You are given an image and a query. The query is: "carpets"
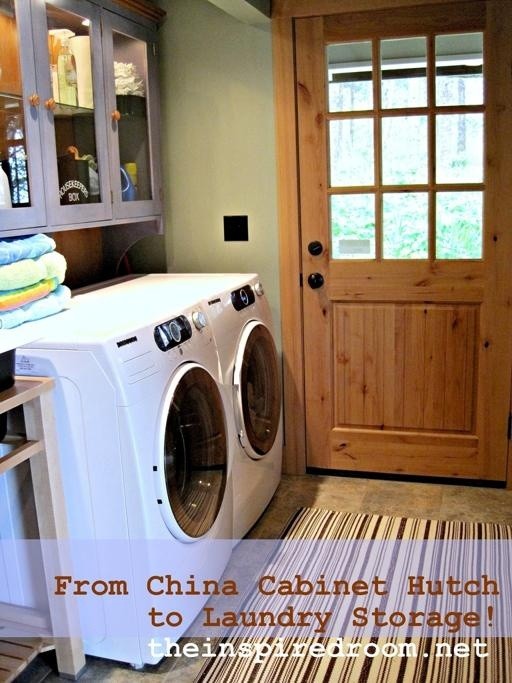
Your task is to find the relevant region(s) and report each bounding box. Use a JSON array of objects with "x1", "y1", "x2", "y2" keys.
[{"x1": 194, "y1": 506, "x2": 512, "y2": 683}]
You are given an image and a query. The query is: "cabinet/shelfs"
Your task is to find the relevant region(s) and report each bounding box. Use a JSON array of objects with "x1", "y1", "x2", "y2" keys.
[{"x1": 0, "y1": 0, "x2": 169, "y2": 238}]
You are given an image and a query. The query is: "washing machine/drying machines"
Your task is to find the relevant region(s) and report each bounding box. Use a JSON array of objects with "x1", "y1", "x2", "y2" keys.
[
  {"x1": 76, "y1": 271, "x2": 285, "y2": 551},
  {"x1": 1, "y1": 298, "x2": 235, "y2": 670}
]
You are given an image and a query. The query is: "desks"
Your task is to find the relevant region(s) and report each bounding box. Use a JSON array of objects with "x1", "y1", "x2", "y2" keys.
[{"x1": 0, "y1": 376, "x2": 88, "y2": 683}]
